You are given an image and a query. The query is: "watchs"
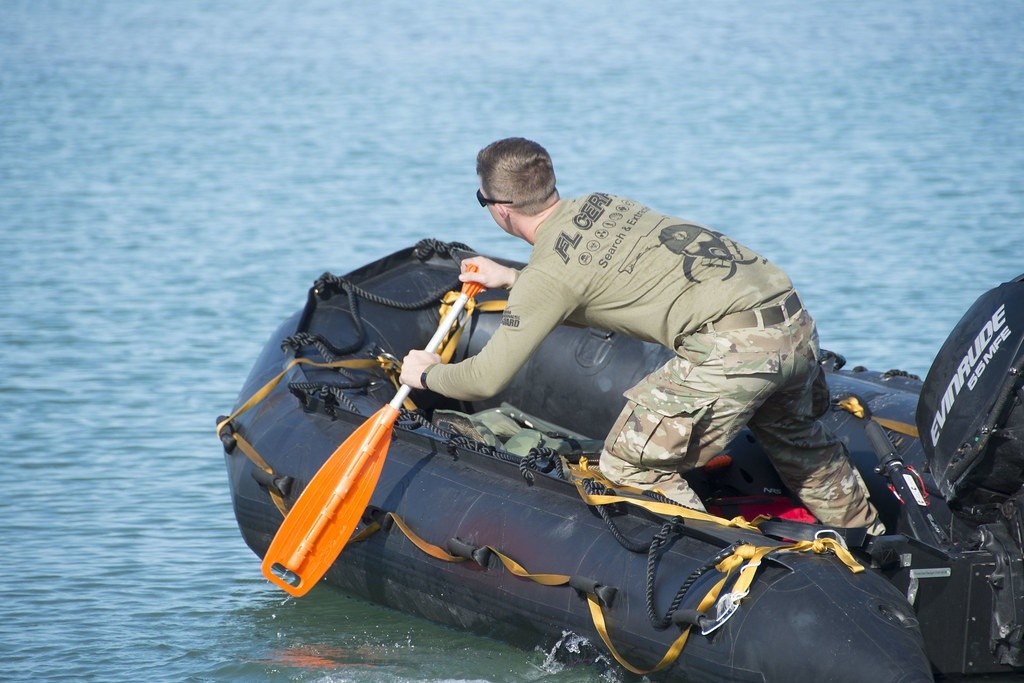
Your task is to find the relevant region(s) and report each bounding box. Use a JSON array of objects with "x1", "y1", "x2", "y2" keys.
[{"x1": 420, "y1": 362, "x2": 443, "y2": 390}]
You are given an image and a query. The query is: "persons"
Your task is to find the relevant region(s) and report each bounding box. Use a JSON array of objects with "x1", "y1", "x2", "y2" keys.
[{"x1": 396, "y1": 135, "x2": 892, "y2": 539}]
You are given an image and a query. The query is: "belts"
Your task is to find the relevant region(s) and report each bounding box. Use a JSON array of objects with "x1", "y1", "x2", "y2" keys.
[{"x1": 697, "y1": 293, "x2": 802, "y2": 334}]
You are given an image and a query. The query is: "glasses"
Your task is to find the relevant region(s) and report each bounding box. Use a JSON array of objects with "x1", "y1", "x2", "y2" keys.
[{"x1": 477, "y1": 189, "x2": 513, "y2": 207}]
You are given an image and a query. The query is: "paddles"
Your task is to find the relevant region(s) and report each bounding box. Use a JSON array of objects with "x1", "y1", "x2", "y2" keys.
[{"x1": 259, "y1": 263, "x2": 485, "y2": 599}]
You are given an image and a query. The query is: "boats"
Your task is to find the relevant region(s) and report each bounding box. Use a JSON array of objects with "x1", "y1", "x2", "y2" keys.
[{"x1": 217, "y1": 238, "x2": 1022, "y2": 683}]
